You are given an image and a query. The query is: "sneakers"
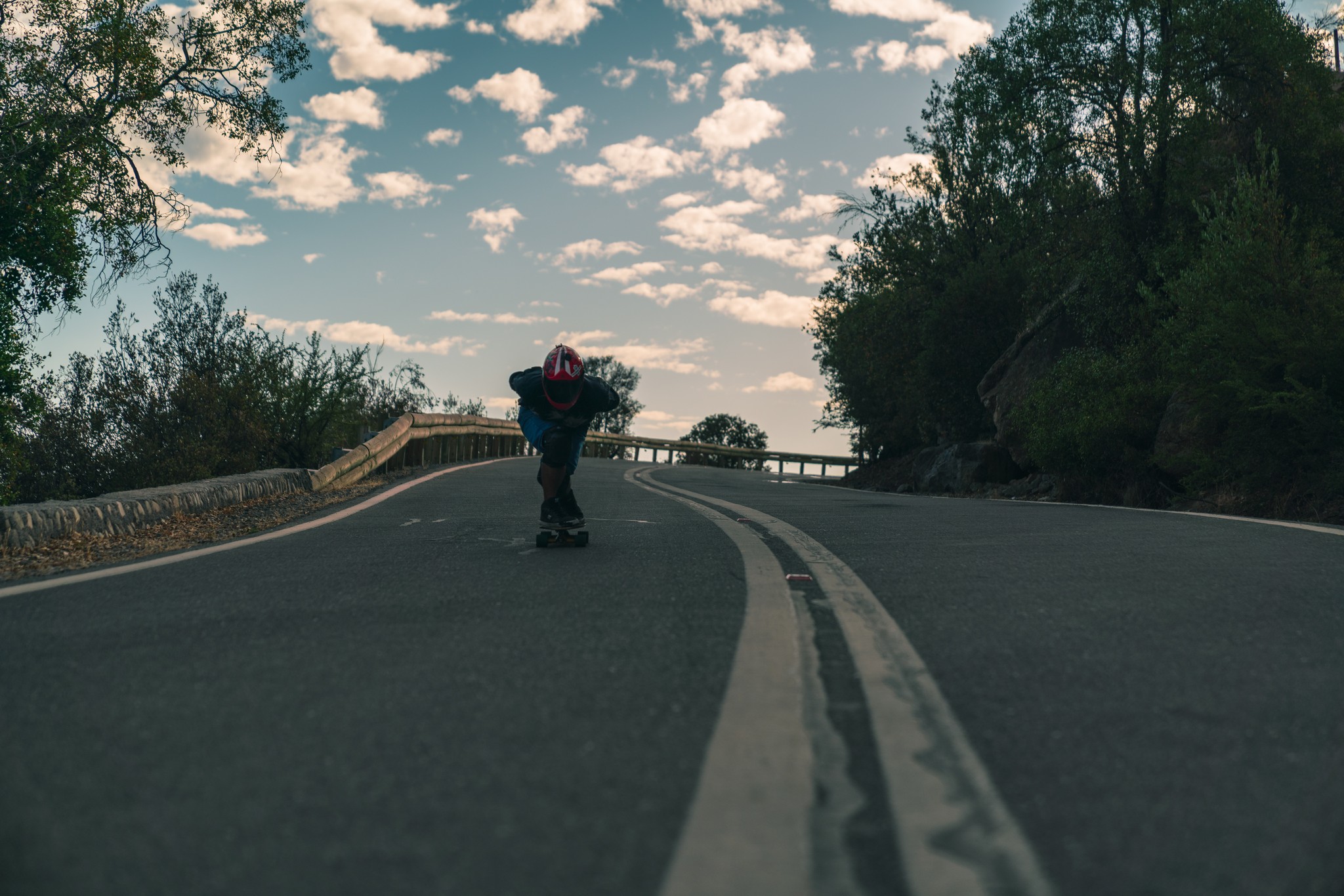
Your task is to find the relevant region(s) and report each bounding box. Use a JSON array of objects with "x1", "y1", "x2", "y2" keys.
[{"x1": 538, "y1": 487, "x2": 587, "y2": 530}]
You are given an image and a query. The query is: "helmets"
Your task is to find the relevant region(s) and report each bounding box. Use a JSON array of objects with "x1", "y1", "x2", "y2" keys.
[{"x1": 543, "y1": 345, "x2": 584, "y2": 379}]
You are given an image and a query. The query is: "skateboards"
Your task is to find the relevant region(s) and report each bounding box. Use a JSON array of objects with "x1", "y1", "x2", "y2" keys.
[{"x1": 536, "y1": 523, "x2": 589, "y2": 547}]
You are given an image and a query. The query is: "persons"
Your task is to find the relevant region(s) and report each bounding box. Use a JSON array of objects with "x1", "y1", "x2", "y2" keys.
[{"x1": 507, "y1": 343, "x2": 620, "y2": 527}]
